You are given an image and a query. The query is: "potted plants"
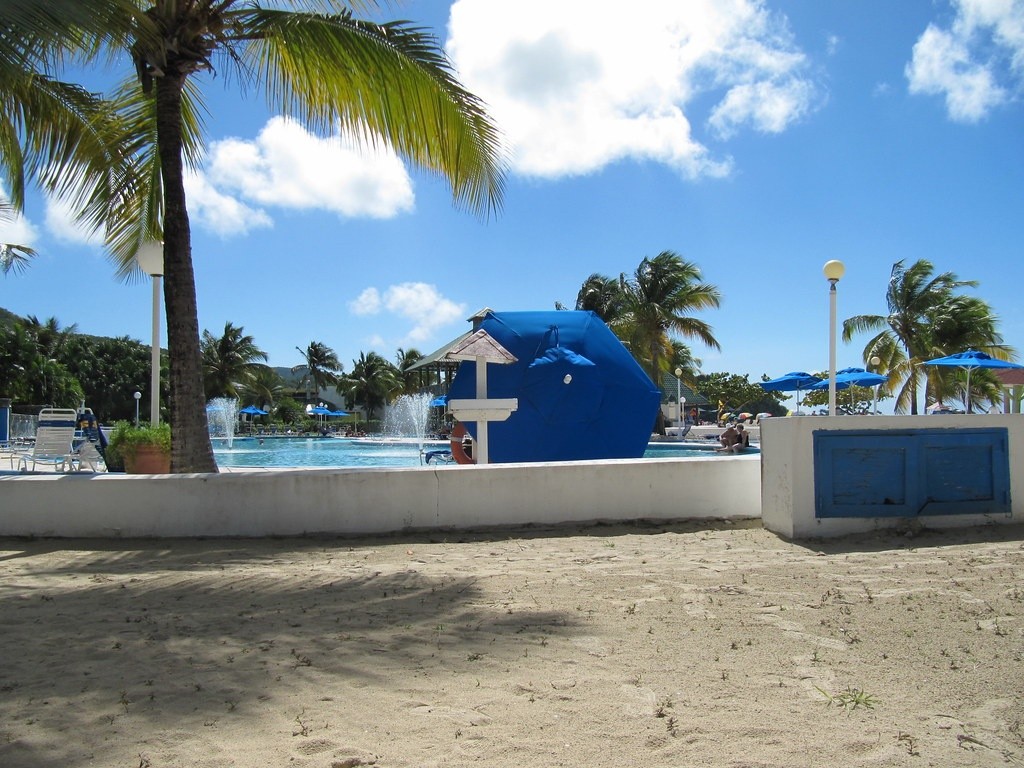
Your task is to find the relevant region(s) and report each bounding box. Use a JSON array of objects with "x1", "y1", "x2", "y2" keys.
[{"x1": 104, "y1": 418, "x2": 171, "y2": 474}]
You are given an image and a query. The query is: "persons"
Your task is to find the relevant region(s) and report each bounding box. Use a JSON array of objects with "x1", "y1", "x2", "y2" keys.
[
  {"x1": 723, "y1": 418, "x2": 753, "y2": 427},
  {"x1": 986, "y1": 402, "x2": 1002, "y2": 414},
  {"x1": 320, "y1": 424, "x2": 364, "y2": 437},
  {"x1": 732, "y1": 424, "x2": 749, "y2": 453},
  {"x1": 439, "y1": 425, "x2": 451, "y2": 434},
  {"x1": 717, "y1": 423, "x2": 738, "y2": 451},
  {"x1": 260, "y1": 438, "x2": 264, "y2": 445},
  {"x1": 700, "y1": 418, "x2": 705, "y2": 425}
]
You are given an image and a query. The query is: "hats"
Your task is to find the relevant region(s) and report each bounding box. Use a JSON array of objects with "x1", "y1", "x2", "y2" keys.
[{"x1": 723, "y1": 423, "x2": 735, "y2": 431}]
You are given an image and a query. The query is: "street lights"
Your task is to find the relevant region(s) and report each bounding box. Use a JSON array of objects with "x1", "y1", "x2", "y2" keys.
[
  {"x1": 680, "y1": 397, "x2": 686, "y2": 426},
  {"x1": 137, "y1": 240, "x2": 164, "y2": 429},
  {"x1": 319, "y1": 402, "x2": 323, "y2": 426},
  {"x1": 871, "y1": 356, "x2": 880, "y2": 415},
  {"x1": 134, "y1": 392, "x2": 141, "y2": 427},
  {"x1": 324, "y1": 404, "x2": 328, "y2": 427},
  {"x1": 823, "y1": 259, "x2": 844, "y2": 416},
  {"x1": 675, "y1": 367, "x2": 682, "y2": 440}
]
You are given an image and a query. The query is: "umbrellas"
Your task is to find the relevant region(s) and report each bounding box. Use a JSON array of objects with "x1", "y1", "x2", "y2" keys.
[
  {"x1": 926, "y1": 401, "x2": 954, "y2": 410},
  {"x1": 720, "y1": 413, "x2": 736, "y2": 421},
  {"x1": 430, "y1": 396, "x2": 447, "y2": 407},
  {"x1": 309, "y1": 406, "x2": 350, "y2": 428},
  {"x1": 758, "y1": 372, "x2": 824, "y2": 415},
  {"x1": 985, "y1": 369, "x2": 1024, "y2": 389},
  {"x1": 813, "y1": 367, "x2": 890, "y2": 415},
  {"x1": 738, "y1": 413, "x2": 753, "y2": 419},
  {"x1": 444, "y1": 311, "x2": 661, "y2": 463},
  {"x1": 914, "y1": 347, "x2": 1024, "y2": 414},
  {"x1": 786, "y1": 410, "x2": 804, "y2": 416},
  {"x1": 756, "y1": 413, "x2": 771, "y2": 420},
  {"x1": 205, "y1": 404, "x2": 269, "y2": 436}
]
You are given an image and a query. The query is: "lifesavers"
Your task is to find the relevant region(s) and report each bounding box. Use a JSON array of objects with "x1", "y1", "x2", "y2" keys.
[{"x1": 451, "y1": 421, "x2": 477, "y2": 464}]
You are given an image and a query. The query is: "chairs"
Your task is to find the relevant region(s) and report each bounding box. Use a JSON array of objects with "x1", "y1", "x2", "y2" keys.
[
  {"x1": 425, "y1": 439, "x2": 472, "y2": 465},
  {"x1": 10, "y1": 408, "x2": 107, "y2": 473},
  {"x1": 668, "y1": 421, "x2": 705, "y2": 441}
]
